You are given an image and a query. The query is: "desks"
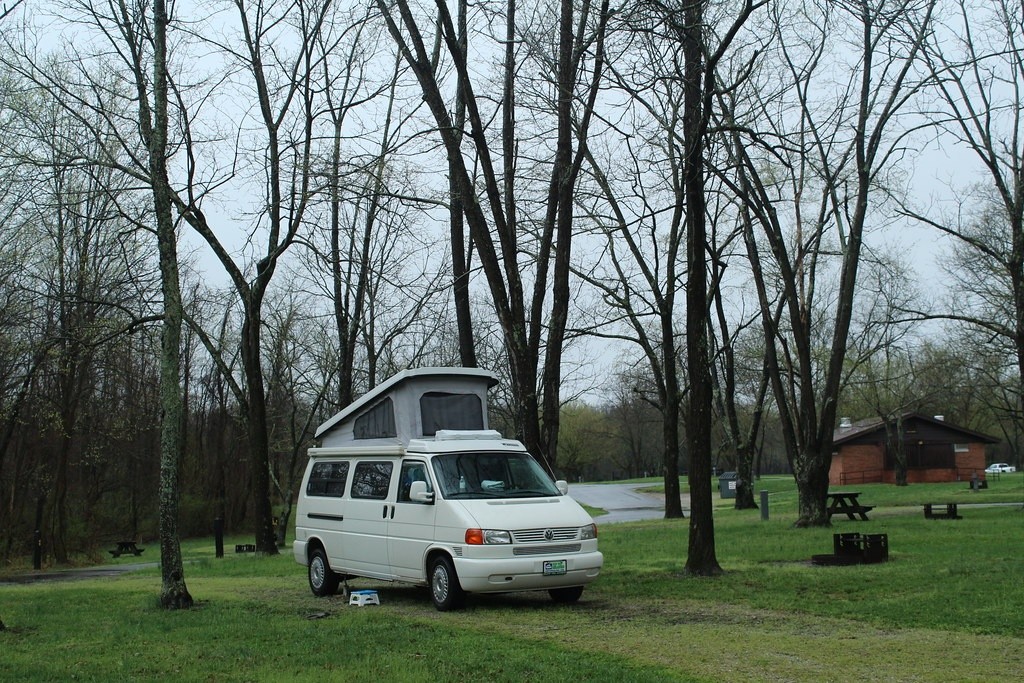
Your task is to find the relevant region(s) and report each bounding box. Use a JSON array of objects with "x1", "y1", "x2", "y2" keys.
[
  {"x1": 827, "y1": 492, "x2": 870, "y2": 521},
  {"x1": 112, "y1": 541, "x2": 142, "y2": 558}
]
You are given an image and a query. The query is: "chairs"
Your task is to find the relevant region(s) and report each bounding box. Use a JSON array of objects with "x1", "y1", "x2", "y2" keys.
[{"x1": 413, "y1": 468, "x2": 426, "y2": 481}]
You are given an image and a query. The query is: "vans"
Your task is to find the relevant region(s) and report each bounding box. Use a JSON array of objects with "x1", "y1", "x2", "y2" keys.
[{"x1": 292, "y1": 366, "x2": 602, "y2": 612}]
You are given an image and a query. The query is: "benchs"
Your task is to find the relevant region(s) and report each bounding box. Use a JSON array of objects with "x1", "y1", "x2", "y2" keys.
[
  {"x1": 137, "y1": 548, "x2": 145, "y2": 553},
  {"x1": 108, "y1": 550, "x2": 116, "y2": 554},
  {"x1": 827, "y1": 505, "x2": 876, "y2": 514}
]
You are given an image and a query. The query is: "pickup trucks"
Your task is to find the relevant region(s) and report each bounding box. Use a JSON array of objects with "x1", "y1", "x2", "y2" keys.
[{"x1": 984, "y1": 463, "x2": 1015, "y2": 473}]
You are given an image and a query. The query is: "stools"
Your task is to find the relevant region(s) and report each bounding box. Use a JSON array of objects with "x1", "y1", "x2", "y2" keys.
[{"x1": 349, "y1": 590, "x2": 381, "y2": 606}]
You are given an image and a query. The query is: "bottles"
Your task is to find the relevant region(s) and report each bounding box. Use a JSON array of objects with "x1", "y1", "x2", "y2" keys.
[{"x1": 459, "y1": 475, "x2": 465, "y2": 493}]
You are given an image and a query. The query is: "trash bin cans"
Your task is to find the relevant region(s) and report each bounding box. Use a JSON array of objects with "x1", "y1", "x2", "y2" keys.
[{"x1": 718, "y1": 471, "x2": 754, "y2": 499}]
